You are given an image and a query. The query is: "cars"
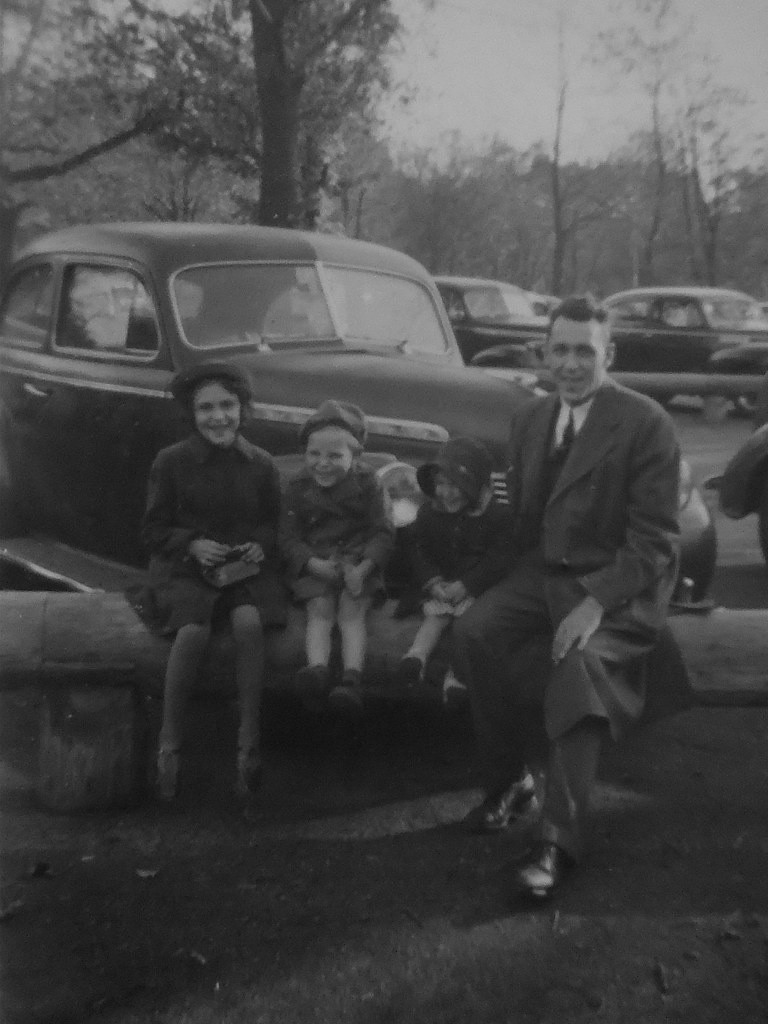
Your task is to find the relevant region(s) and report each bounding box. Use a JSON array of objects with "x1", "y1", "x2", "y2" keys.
[
  {"x1": 429, "y1": 273, "x2": 554, "y2": 368},
  {"x1": 595, "y1": 286, "x2": 768, "y2": 420},
  {"x1": 1, "y1": 221, "x2": 727, "y2": 620}
]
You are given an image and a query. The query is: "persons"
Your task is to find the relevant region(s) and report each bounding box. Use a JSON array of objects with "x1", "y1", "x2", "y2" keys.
[
  {"x1": 399, "y1": 437, "x2": 507, "y2": 712},
  {"x1": 449, "y1": 295, "x2": 682, "y2": 896},
  {"x1": 275, "y1": 400, "x2": 395, "y2": 711},
  {"x1": 124, "y1": 364, "x2": 282, "y2": 797}
]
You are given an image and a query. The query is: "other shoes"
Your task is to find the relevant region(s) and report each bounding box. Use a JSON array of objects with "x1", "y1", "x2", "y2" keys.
[
  {"x1": 232, "y1": 750, "x2": 265, "y2": 790},
  {"x1": 439, "y1": 675, "x2": 468, "y2": 708},
  {"x1": 329, "y1": 677, "x2": 369, "y2": 721},
  {"x1": 149, "y1": 740, "x2": 187, "y2": 797},
  {"x1": 394, "y1": 652, "x2": 427, "y2": 683},
  {"x1": 293, "y1": 666, "x2": 328, "y2": 716}
]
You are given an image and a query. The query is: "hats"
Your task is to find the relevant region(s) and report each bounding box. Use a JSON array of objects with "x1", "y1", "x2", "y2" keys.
[
  {"x1": 301, "y1": 399, "x2": 365, "y2": 445},
  {"x1": 415, "y1": 437, "x2": 493, "y2": 510},
  {"x1": 164, "y1": 358, "x2": 247, "y2": 408}
]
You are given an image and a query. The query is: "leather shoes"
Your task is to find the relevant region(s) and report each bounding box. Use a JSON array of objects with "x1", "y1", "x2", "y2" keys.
[
  {"x1": 510, "y1": 840, "x2": 562, "y2": 891},
  {"x1": 470, "y1": 771, "x2": 537, "y2": 836}
]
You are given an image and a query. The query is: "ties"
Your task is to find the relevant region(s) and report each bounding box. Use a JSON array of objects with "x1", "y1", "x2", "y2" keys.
[{"x1": 538, "y1": 417, "x2": 574, "y2": 527}]
